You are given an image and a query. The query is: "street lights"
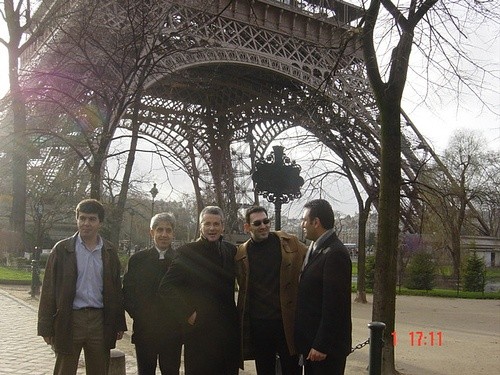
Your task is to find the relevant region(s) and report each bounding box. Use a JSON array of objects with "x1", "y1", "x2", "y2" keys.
[{"x1": 149, "y1": 182, "x2": 159, "y2": 247}]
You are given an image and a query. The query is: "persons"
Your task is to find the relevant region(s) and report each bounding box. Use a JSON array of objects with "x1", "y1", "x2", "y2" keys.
[
  {"x1": 121, "y1": 213, "x2": 182, "y2": 375},
  {"x1": 234, "y1": 207, "x2": 308, "y2": 375},
  {"x1": 158, "y1": 205, "x2": 239, "y2": 374},
  {"x1": 293, "y1": 199, "x2": 353, "y2": 374},
  {"x1": 37, "y1": 200, "x2": 128, "y2": 375}
]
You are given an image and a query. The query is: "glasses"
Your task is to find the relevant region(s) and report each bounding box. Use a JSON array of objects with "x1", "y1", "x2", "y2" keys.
[{"x1": 247, "y1": 219, "x2": 270, "y2": 226}]
